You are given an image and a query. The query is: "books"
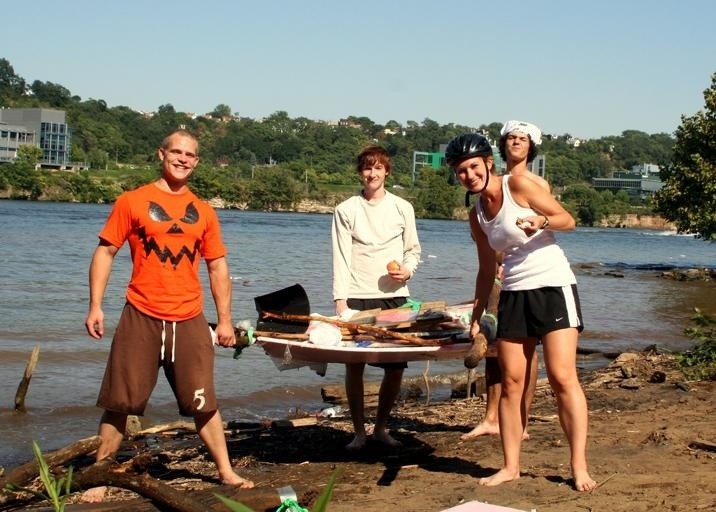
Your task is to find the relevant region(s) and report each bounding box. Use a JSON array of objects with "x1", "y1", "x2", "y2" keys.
[{"x1": 340, "y1": 302, "x2": 475, "y2": 342}]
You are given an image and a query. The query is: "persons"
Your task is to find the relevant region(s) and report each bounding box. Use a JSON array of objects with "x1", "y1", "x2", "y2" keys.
[
  {"x1": 460, "y1": 118, "x2": 553, "y2": 440},
  {"x1": 443, "y1": 132, "x2": 597, "y2": 490},
  {"x1": 81, "y1": 129, "x2": 255, "y2": 502},
  {"x1": 333, "y1": 146, "x2": 421, "y2": 448}
]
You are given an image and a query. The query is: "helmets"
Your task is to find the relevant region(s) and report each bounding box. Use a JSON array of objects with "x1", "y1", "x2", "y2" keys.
[{"x1": 446, "y1": 133, "x2": 492, "y2": 166}]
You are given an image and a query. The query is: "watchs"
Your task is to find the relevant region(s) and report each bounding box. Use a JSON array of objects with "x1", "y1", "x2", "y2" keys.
[{"x1": 539, "y1": 214, "x2": 550, "y2": 230}]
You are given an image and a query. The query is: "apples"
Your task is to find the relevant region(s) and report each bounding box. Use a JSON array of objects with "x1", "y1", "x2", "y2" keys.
[{"x1": 387, "y1": 260, "x2": 399, "y2": 273}]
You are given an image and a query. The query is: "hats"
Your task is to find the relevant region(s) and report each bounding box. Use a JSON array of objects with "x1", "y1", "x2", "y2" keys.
[{"x1": 500, "y1": 121, "x2": 543, "y2": 145}]
